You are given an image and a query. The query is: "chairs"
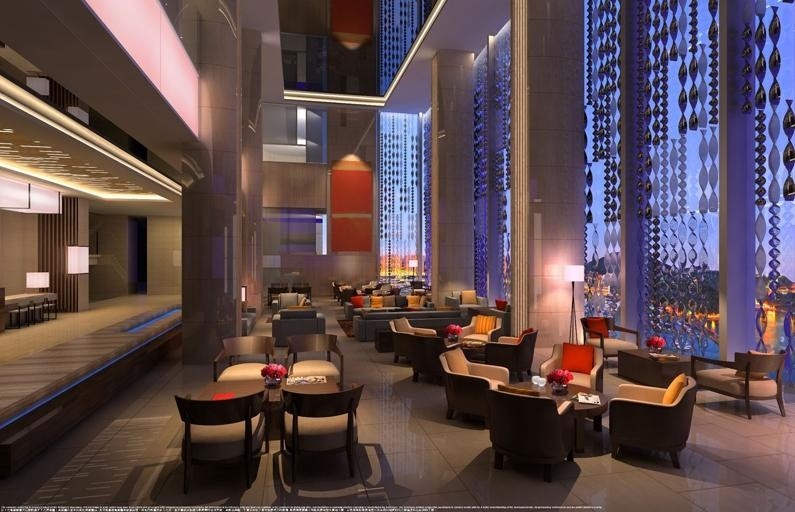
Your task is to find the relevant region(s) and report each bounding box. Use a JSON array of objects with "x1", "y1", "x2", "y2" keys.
[
  {"x1": 30, "y1": 295, "x2": 44, "y2": 324},
  {"x1": 266, "y1": 277, "x2": 537, "y2": 381},
  {"x1": 540, "y1": 343, "x2": 603, "y2": 432},
  {"x1": 44, "y1": 294, "x2": 58, "y2": 320},
  {"x1": 609, "y1": 373, "x2": 697, "y2": 470},
  {"x1": 278, "y1": 385, "x2": 365, "y2": 480},
  {"x1": 692, "y1": 349, "x2": 789, "y2": 419},
  {"x1": 213, "y1": 335, "x2": 273, "y2": 382},
  {"x1": 175, "y1": 392, "x2": 271, "y2": 491},
  {"x1": 284, "y1": 335, "x2": 344, "y2": 386},
  {"x1": 581, "y1": 317, "x2": 640, "y2": 365},
  {"x1": 10, "y1": 297, "x2": 32, "y2": 326},
  {"x1": 440, "y1": 348, "x2": 510, "y2": 427},
  {"x1": 488, "y1": 389, "x2": 574, "y2": 484}
]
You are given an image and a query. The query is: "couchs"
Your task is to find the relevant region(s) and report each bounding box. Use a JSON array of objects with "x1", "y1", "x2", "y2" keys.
[{"x1": 241, "y1": 305, "x2": 257, "y2": 335}]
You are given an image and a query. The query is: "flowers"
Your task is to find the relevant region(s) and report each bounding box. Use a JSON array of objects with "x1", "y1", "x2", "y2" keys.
[
  {"x1": 260, "y1": 363, "x2": 287, "y2": 378},
  {"x1": 547, "y1": 368, "x2": 574, "y2": 384},
  {"x1": 645, "y1": 336, "x2": 666, "y2": 347}
]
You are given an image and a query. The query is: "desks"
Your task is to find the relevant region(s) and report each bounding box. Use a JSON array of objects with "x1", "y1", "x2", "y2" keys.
[
  {"x1": 498, "y1": 383, "x2": 609, "y2": 452},
  {"x1": 0, "y1": 292, "x2": 57, "y2": 330},
  {"x1": 193, "y1": 381, "x2": 355, "y2": 413},
  {"x1": 618, "y1": 348, "x2": 697, "y2": 386}
]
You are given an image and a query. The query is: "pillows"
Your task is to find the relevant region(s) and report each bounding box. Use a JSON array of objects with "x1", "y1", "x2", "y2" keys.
[
  {"x1": 498, "y1": 385, "x2": 540, "y2": 396},
  {"x1": 587, "y1": 318, "x2": 609, "y2": 339},
  {"x1": 562, "y1": 342, "x2": 595, "y2": 374},
  {"x1": 735, "y1": 350, "x2": 776, "y2": 378},
  {"x1": 564, "y1": 265, "x2": 584, "y2": 345},
  {"x1": 663, "y1": 373, "x2": 687, "y2": 405}
]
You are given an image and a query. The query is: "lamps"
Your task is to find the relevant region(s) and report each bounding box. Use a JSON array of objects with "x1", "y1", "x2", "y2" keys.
[
  {"x1": 26, "y1": 272, "x2": 49, "y2": 288},
  {"x1": 240, "y1": 285, "x2": 248, "y2": 303},
  {"x1": 409, "y1": 260, "x2": 419, "y2": 280},
  {"x1": 67, "y1": 245, "x2": 89, "y2": 274},
  {"x1": 0, "y1": 177, "x2": 63, "y2": 215}
]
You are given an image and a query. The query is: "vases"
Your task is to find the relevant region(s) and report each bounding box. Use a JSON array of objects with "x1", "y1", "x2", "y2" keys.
[
  {"x1": 265, "y1": 377, "x2": 283, "y2": 388},
  {"x1": 650, "y1": 346, "x2": 658, "y2": 352},
  {"x1": 551, "y1": 384, "x2": 568, "y2": 395}
]
